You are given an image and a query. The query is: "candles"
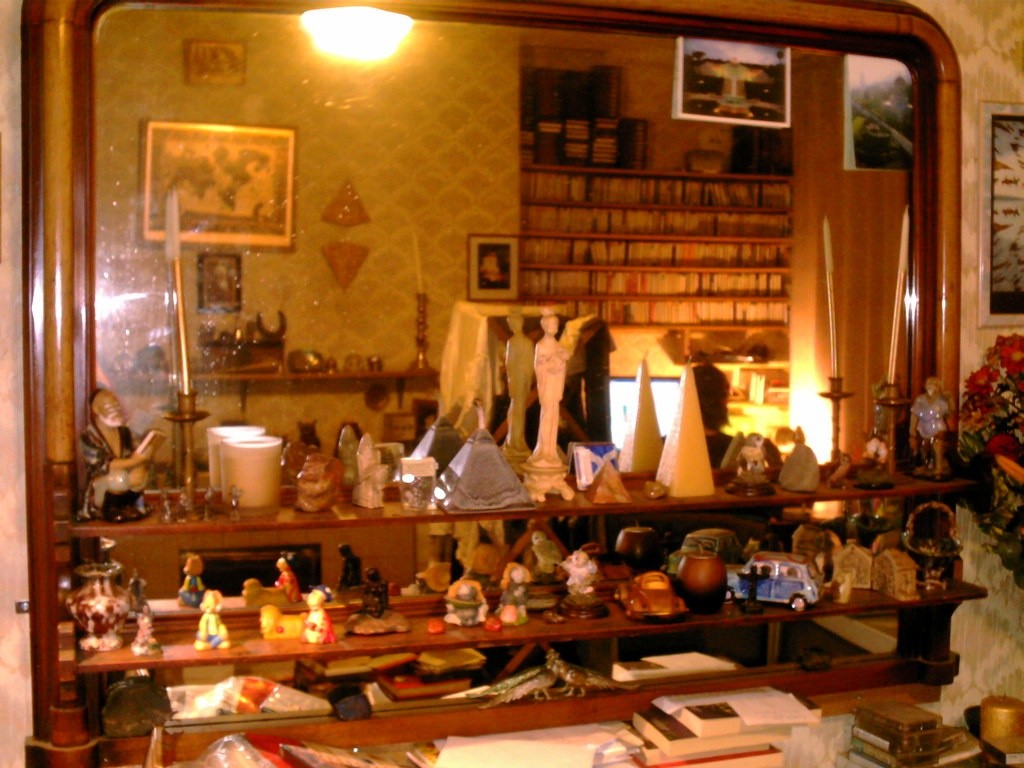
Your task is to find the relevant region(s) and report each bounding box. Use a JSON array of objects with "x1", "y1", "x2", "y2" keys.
[
  {"x1": 886, "y1": 203, "x2": 911, "y2": 386},
  {"x1": 164, "y1": 189, "x2": 180, "y2": 386},
  {"x1": 411, "y1": 225, "x2": 426, "y2": 293},
  {"x1": 172, "y1": 183, "x2": 192, "y2": 394},
  {"x1": 822, "y1": 214, "x2": 843, "y2": 378}
]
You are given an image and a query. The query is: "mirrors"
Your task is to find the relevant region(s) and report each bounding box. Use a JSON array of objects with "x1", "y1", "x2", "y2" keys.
[{"x1": 23, "y1": 0, "x2": 963, "y2": 768}]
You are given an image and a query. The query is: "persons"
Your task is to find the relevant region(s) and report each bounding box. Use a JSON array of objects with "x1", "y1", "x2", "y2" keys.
[
  {"x1": 505, "y1": 314, "x2": 534, "y2": 454},
  {"x1": 337, "y1": 543, "x2": 360, "y2": 591},
  {"x1": 528, "y1": 314, "x2": 568, "y2": 469},
  {"x1": 295, "y1": 454, "x2": 340, "y2": 512},
  {"x1": 355, "y1": 567, "x2": 388, "y2": 617},
  {"x1": 273, "y1": 552, "x2": 303, "y2": 604},
  {"x1": 303, "y1": 584, "x2": 337, "y2": 644},
  {"x1": 662, "y1": 365, "x2": 733, "y2": 469},
  {"x1": 908, "y1": 377, "x2": 956, "y2": 478},
  {"x1": 177, "y1": 554, "x2": 209, "y2": 609},
  {"x1": 193, "y1": 588, "x2": 230, "y2": 650},
  {"x1": 444, "y1": 550, "x2": 598, "y2": 627},
  {"x1": 76, "y1": 387, "x2": 153, "y2": 522}
]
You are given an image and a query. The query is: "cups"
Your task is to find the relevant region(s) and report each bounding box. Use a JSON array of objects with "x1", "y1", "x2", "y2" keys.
[
  {"x1": 220, "y1": 436, "x2": 283, "y2": 516},
  {"x1": 206, "y1": 426, "x2": 266, "y2": 508},
  {"x1": 400, "y1": 457, "x2": 436, "y2": 512}
]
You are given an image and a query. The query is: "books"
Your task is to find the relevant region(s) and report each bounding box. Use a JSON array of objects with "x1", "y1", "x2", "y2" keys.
[
  {"x1": 518, "y1": 67, "x2": 792, "y2": 327},
  {"x1": 618, "y1": 685, "x2": 821, "y2": 767},
  {"x1": 612, "y1": 654, "x2": 740, "y2": 682},
  {"x1": 290, "y1": 647, "x2": 489, "y2": 703}
]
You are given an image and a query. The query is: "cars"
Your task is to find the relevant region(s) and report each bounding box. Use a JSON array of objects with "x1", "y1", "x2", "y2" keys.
[{"x1": 725, "y1": 550, "x2": 824, "y2": 613}]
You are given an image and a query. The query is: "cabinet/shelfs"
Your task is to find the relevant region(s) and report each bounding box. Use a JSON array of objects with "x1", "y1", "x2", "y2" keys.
[
  {"x1": 70, "y1": 477, "x2": 990, "y2": 686},
  {"x1": 511, "y1": 161, "x2": 797, "y2": 331}
]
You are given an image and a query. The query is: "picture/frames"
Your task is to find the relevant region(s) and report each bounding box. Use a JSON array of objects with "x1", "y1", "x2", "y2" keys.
[
  {"x1": 671, "y1": 36, "x2": 794, "y2": 130},
  {"x1": 196, "y1": 252, "x2": 242, "y2": 314},
  {"x1": 466, "y1": 233, "x2": 523, "y2": 302},
  {"x1": 184, "y1": 39, "x2": 248, "y2": 85},
  {"x1": 136, "y1": 117, "x2": 299, "y2": 254},
  {"x1": 978, "y1": 99, "x2": 1024, "y2": 328}
]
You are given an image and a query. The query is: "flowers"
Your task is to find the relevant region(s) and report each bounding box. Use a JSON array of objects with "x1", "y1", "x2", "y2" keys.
[{"x1": 958, "y1": 332, "x2": 1024, "y2": 589}]
